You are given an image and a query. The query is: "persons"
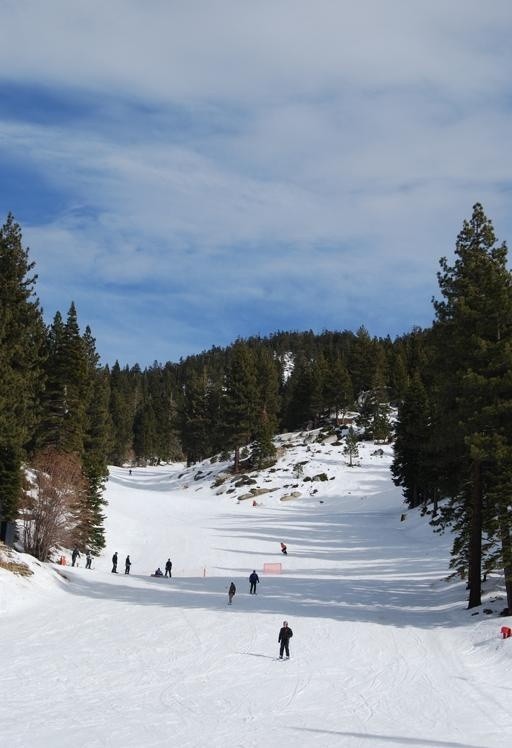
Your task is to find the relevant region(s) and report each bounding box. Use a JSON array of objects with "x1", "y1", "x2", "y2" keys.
[
  {"x1": 250, "y1": 569, "x2": 261, "y2": 595},
  {"x1": 227, "y1": 582, "x2": 238, "y2": 604},
  {"x1": 277, "y1": 620, "x2": 294, "y2": 661},
  {"x1": 281, "y1": 541, "x2": 289, "y2": 556},
  {"x1": 71, "y1": 545, "x2": 174, "y2": 580}
]
